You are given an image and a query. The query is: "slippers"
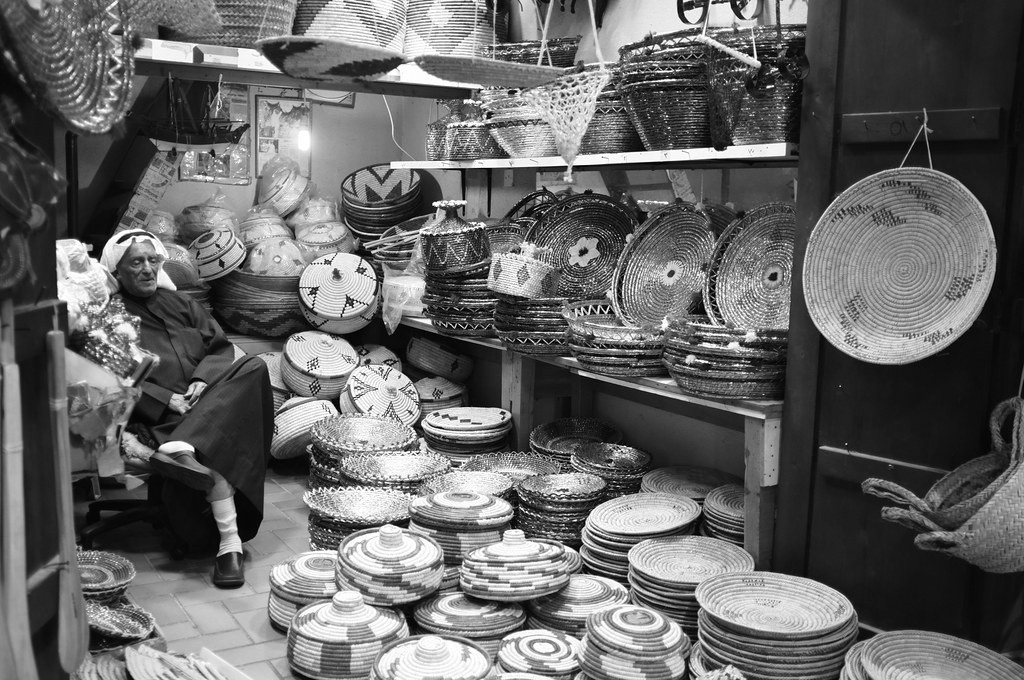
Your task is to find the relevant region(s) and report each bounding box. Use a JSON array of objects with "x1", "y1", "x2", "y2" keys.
[
  {"x1": 213, "y1": 551, "x2": 245, "y2": 586},
  {"x1": 149, "y1": 450, "x2": 215, "y2": 491}
]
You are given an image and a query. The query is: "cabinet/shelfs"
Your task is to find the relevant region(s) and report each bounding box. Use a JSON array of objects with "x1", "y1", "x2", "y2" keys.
[{"x1": 59, "y1": 54, "x2": 799, "y2": 571}]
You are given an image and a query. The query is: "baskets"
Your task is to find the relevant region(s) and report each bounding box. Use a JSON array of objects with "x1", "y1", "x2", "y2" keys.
[{"x1": 1, "y1": 1, "x2": 1024, "y2": 680}]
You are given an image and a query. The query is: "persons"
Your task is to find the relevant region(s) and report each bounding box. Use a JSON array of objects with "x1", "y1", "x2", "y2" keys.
[{"x1": 67, "y1": 228, "x2": 274, "y2": 587}]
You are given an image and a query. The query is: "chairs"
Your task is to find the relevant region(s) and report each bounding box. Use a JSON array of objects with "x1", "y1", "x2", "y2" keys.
[{"x1": 80, "y1": 393, "x2": 218, "y2": 562}]
